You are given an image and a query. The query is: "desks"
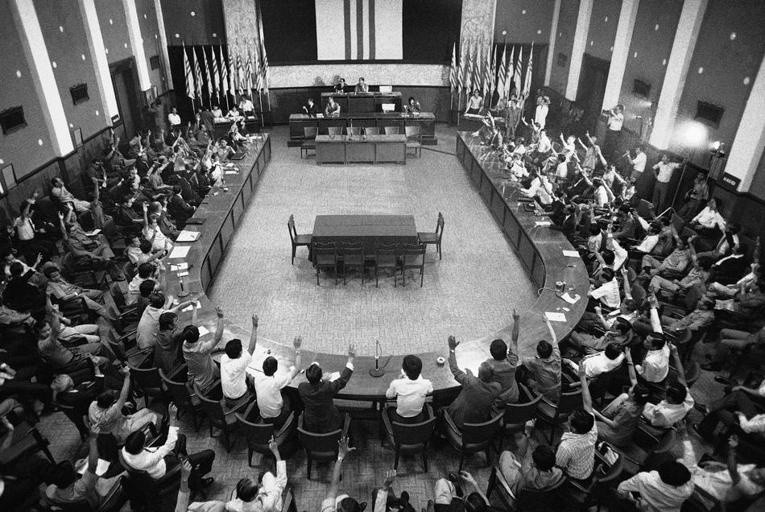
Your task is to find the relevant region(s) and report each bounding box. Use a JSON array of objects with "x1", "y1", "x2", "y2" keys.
[{"x1": 320, "y1": 92, "x2": 402, "y2": 116}]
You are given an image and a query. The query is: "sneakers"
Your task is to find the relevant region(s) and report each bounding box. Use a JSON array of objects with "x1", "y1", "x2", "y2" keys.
[{"x1": 700, "y1": 353, "x2": 721, "y2": 371}]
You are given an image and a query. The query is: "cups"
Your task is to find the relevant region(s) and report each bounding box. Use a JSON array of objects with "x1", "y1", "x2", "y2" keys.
[{"x1": 556, "y1": 282, "x2": 563, "y2": 291}]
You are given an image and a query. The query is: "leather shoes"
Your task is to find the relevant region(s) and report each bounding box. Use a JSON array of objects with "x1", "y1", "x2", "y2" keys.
[
  {"x1": 400, "y1": 491, "x2": 409, "y2": 504},
  {"x1": 694, "y1": 401, "x2": 708, "y2": 417},
  {"x1": 200, "y1": 477, "x2": 214, "y2": 489},
  {"x1": 448, "y1": 473, "x2": 463, "y2": 498},
  {"x1": 358, "y1": 502, "x2": 367, "y2": 512}
]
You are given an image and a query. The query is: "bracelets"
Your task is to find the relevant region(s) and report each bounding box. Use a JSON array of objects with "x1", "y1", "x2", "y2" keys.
[
  {"x1": 296, "y1": 351, "x2": 300, "y2": 354},
  {"x1": 450, "y1": 350, "x2": 454, "y2": 353},
  {"x1": 337, "y1": 457, "x2": 344, "y2": 463}
]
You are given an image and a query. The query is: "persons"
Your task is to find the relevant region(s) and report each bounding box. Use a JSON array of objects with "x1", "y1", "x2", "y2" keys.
[
  {"x1": 225, "y1": 435, "x2": 288, "y2": 512},
  {"x1": 437, "y1": 335, "x2": 502, "y2": 432},
  {"x1": 434, "y1": 469, "x2": 491, "y2": 512},
  {"x1": 372, "y1": 469, "x2": 417, "y2": 512},
  {"x1": 303, "y1": 77, "x2": 369, "y2": 116},
  {"x1": 298, "y1": 343, "x2": 356, "y2": 434},
  {"x1": 386, "y1": 355, "x2": 434, "y2": 424},
  {"x1": 254, "y1": 335, "x2": 303, "y2": 430},
  {"x1": 464, "y1": 89, "x2": 765, "y2": 512},
  {"x1": 0, "y1": 95, "x2": 258, "y2": 512},
  {"x1": 405, "y1": 96, "x2": 421, "y2": 113},
  {"x1": 321, "y1": 435, "x2": 368, "y2": 511}
]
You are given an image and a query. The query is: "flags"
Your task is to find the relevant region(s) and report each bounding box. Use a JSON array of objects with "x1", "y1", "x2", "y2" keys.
[
  {"x1": 183, "y1": 43, "x2": 269, "y2": 98},
  {"x1": 449, "y1": 43, "x2": 533, "y2": 100}
]
